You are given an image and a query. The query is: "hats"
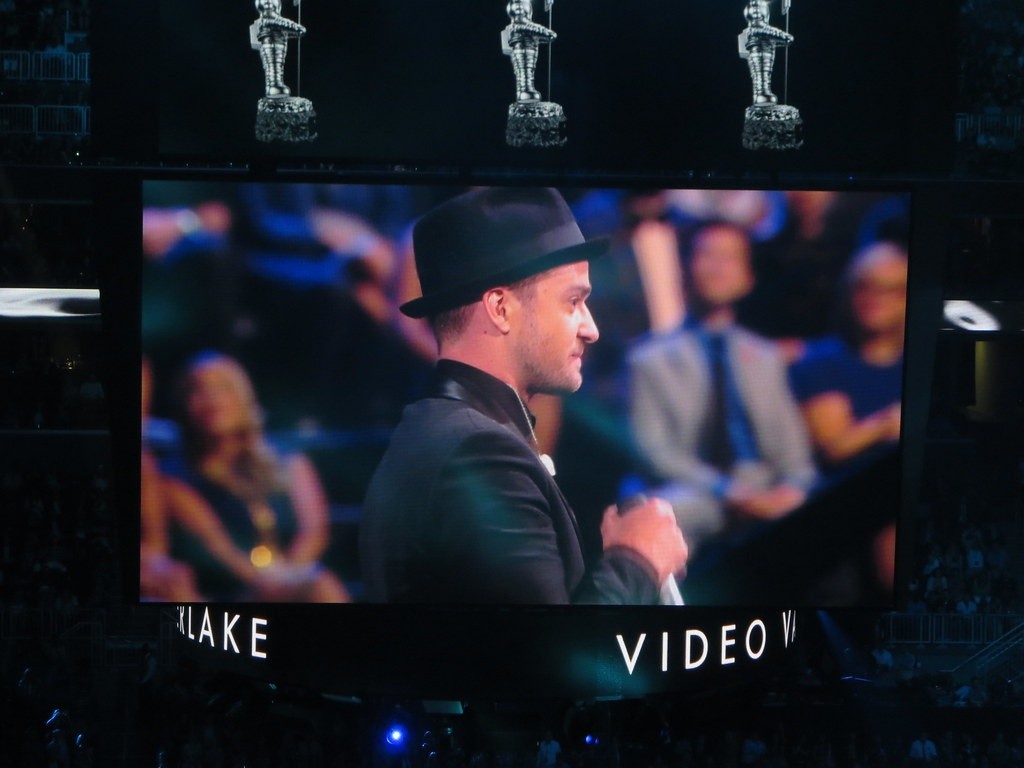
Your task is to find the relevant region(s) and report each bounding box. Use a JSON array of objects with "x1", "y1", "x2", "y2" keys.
[{"x1": 398, "y1": 180, "x2": 613, "y2": 321}]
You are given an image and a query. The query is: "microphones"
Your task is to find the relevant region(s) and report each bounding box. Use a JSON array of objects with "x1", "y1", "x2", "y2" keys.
[{"x1": 621, "y1": 493, "x2": 684, "y2": 607}]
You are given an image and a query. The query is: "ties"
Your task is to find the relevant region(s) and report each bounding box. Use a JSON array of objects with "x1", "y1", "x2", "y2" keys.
[{"x1": 696, "y1": 334, "x2": 739, "y2": 480}]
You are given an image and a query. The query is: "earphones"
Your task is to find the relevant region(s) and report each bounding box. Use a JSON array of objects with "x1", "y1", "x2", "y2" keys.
[{"x1": 540, "y1": 453, "x2": 556, "y2": 477}]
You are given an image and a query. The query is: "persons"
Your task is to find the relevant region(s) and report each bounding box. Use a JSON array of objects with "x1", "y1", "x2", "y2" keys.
[
  {"x1": 942, "y1": 674, "x2": 993, "y2": 703},
  {"x1": 536, "y1": 730, "x2": 562, "y2": 768},
  {"x1": 960, "y1": 128, "x2": 997, "y2": 150},
  {"x1": 914, "y1": 519, "x2": 1016, "y2": 614},
  {"x1": 356, "y1": 186, "x2": 689, "y2": 603},
  {"x1": 142, "y1": 178, "x2": 911, "y2": 604},
  {"x1": 909, "y1": 734, "x2": 937, "y2": 762},
  {"x1": 563, "y1": 698, "x2": 1024, "y2": 768},
  {"x1": 872, "y1": 641, "x2": 915, "y2": 687},
  {"x1": 18, "y1": 641, "x2": 536, "y2": 768},
  {"x1": 741, "y1": 731, "x2": 767, "y2": 764},
  {"x1": 964, "y1": 209, "x2": 991, "y2": 238}
]
